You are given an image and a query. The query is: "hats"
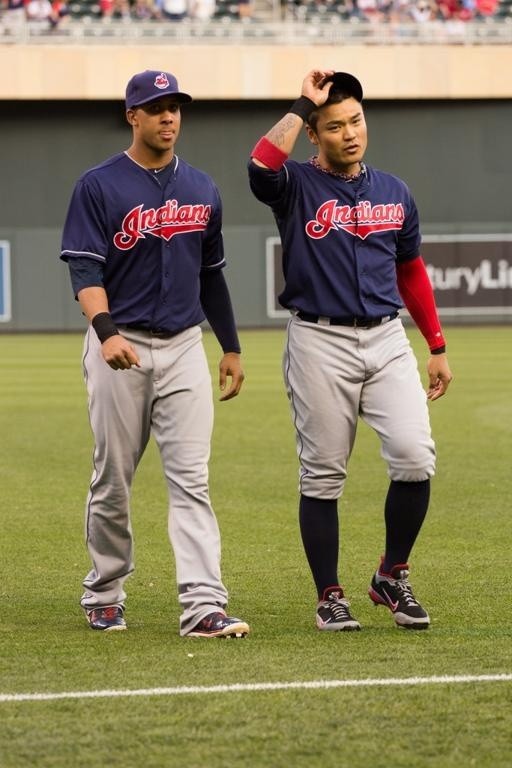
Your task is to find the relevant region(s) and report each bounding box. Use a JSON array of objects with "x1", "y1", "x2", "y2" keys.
[
  {"x1": 125, "y1": 71, "x2": 193, "y2": 111},
  {"x1": 318, "y1": 71, "x2": 363, "y2": 103}
]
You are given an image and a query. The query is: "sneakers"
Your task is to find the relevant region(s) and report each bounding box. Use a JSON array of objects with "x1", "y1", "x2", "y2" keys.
[
  {"x1": 85, "y1": 607, "x2": 127, "y2": 632},
  {"x1": 315, "y1": 585, "x2": 363, "y2": 631},
  {"x1": 185, "y1": 612, "x2": 249, "y2": 639},
  {"x1": 367, "y1": 557, "x2": 431, "y2": 629}
]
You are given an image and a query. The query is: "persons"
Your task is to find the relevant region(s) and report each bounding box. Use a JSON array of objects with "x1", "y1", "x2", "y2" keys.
[
  {"x1": 247, "y1": 68, "x2": 451, "y2": 630},
  {"x1": 60, "y1": 70, "x2": 250, "y2": 639}
]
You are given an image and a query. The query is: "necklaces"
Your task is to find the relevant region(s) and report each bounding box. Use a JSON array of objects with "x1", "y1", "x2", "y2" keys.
[{"x1": 310, "y1": 155, "x2": 367, "y2": 179}]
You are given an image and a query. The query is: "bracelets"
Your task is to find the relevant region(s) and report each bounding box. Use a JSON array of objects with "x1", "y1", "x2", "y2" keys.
[
  {"x1": 288, "y1": 95, "x2": 318, "y2": 123},
  {"x1": 432, "y1": 345, "x2": 446, "y2": 355},
  {"x1": 92, "y1": 313, "x2": 120, "y2": 345}
]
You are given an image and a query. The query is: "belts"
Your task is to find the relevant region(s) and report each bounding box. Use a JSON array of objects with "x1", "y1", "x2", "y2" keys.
[
  {"x1": 297, "y1": 311, "x2": 399, "y2": 326},
  {"x1": 126, "y1": 326, "x2": 168, "y2": 339}
]
